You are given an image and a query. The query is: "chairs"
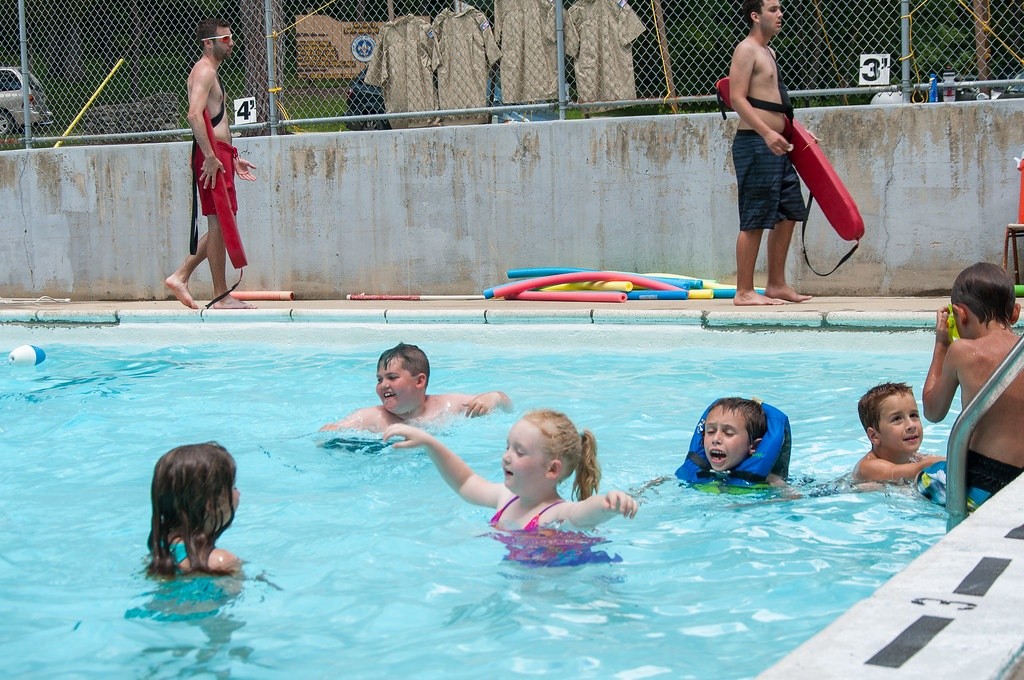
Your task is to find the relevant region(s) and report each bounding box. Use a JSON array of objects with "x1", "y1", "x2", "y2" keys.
[{"x1": 1003, "y1": 156, "x2": 1024, "y2": 282}]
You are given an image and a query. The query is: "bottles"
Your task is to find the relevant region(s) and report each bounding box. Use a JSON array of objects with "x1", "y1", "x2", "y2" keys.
[
  {"x1": 929, "y1": 74, "x2": 938, "y2": 103},
  {"x1": 943, "y1": 65, "x2": 956, "y2": 102}
]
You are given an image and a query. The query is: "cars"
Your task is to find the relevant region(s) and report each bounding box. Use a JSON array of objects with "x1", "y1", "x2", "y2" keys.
[
  {"x1": 344, "y1": 66, "x2": 396, "y2": 130},
  {"x1": 0, "y1": 65, "x2": 53, "y2": 136}
]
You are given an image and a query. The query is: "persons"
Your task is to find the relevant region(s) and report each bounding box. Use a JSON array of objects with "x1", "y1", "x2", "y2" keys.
[
  {"x1": 729, "y1": 0, "x2": 812, "y2": 306},
  {"x1": 146, "y1": 441, "x2": 241, "y2": 575},
  {"x1": 703, "y1": 397, "x2": 784, "y2": 486},
  {"x1": 915, "y1": 261, "x2": 1024, "y2": 512},
  {"x1": 319, "y1": 341, "x2": 515, "y2": 433},
  {"x1": 853, "y1": 381, "x2": 947, "y2": 482},
  {"x1": 383, "y1": 410, "x2": 638, "y2": 533},
  {"x1": 165, "y1": 18, "x2": 258, "y2": 309}
]
